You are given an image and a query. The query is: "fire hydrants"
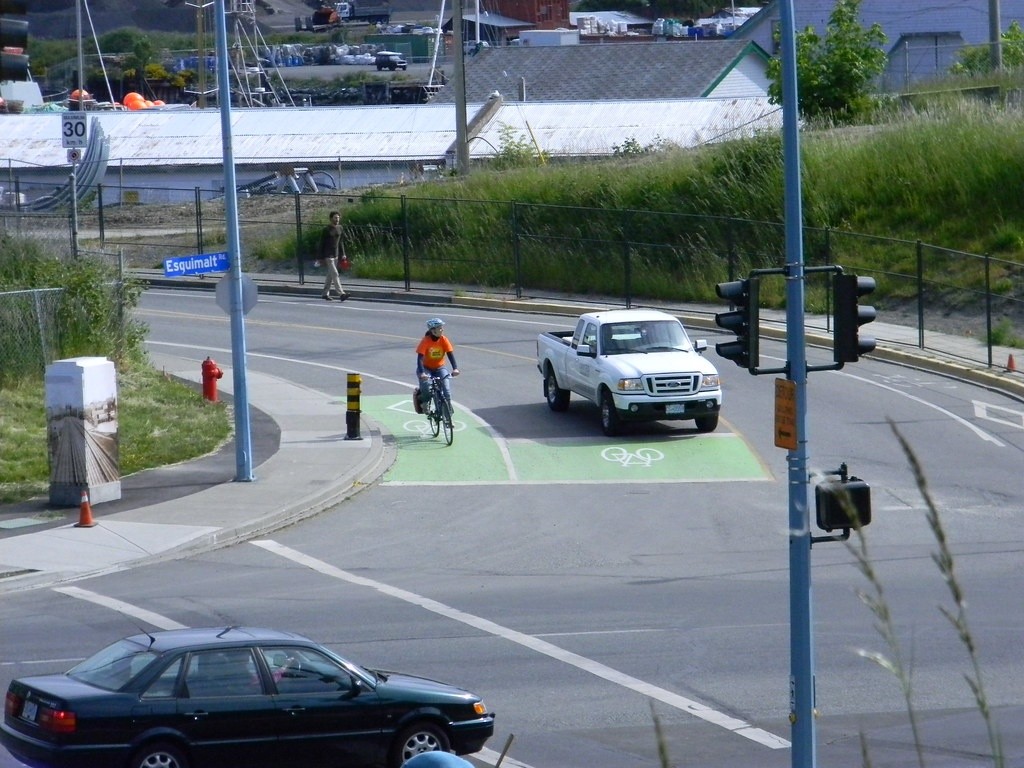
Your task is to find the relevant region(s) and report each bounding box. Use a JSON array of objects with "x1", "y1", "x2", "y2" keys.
[{"x1": 200, "y1": 356, "x2": 224, "y2": 402}]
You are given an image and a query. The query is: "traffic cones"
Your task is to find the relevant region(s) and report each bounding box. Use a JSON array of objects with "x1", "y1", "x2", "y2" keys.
[
  {"x1": 1004, "y1": 353, "x2": 1017, "y2": 373},
  {"x1": 74, "y1": 492, "x2": 99, "y2": 528}
]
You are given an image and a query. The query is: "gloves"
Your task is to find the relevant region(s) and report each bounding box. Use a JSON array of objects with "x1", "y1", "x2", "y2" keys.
[
  {"x1": 420, "y1": 373, "x2": 429, "y2": 382},
  {"x1": 452, "y1": 368, "x2": 459, "y2": 376}
]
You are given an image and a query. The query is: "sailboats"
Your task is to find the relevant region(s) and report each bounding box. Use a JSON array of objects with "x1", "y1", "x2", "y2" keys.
[{"x1": 422, "y1": 0, "x2": 513, "y2": 99}]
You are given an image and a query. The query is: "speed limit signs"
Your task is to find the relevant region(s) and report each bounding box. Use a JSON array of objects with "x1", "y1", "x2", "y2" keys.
[{"x1": 61, "y1": 112, "x2": 89, "y2": 148}]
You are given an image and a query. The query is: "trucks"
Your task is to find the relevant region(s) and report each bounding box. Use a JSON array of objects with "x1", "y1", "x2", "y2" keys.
[{"x1": 334, "y1": 3, "x2": 392, "y2": 24}]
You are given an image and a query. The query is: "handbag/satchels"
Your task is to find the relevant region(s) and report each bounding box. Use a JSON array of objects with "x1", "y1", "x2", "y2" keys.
[{"x1": 414, "y1": 388, "x2": 421, "y2": 413}]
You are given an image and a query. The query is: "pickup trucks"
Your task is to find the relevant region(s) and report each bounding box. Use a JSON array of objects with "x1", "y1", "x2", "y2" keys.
[{"x1": 536, "y1": 308, "x2": 722, "y2": 437}]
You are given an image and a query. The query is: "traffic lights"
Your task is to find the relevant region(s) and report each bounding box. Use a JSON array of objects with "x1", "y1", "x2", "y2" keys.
[
  {"x1": 832, "y1": 273, "x2": 877, "y2": 362},
  {"x1": 714, "y1": 280, "x2": 760, "y2": 367},
  {"x1": 815, "y1": 480, "x2": 872, "y2": 533}
]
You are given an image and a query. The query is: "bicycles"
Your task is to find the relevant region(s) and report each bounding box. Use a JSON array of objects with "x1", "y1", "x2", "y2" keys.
[{"x1": 419, "y1": 373, "x2": 455, "y2": 446}]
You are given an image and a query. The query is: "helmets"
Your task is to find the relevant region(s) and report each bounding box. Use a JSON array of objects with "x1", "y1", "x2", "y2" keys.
[{"x1": 426, "y1": 318, "x2": 445, "y2": 330}]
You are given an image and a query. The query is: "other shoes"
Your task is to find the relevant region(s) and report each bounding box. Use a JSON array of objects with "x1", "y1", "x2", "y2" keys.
[
  {"x1": 420, "y1": 401, "x2": 431, "y2": 415},
  {"x1": 322, "y1": 295, "x2": 333, "y2": 300},
  {"x1": 341, "y1": 294, "x2": 347, "y2": 301}
]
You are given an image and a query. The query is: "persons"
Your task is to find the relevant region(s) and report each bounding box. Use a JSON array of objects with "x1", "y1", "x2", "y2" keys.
[
  {"x1": 415, "y1": 318, "x2": 458, "y2": 428},
  {"x1": 316, "y1": 211, "x2": 350, "y2": 302},
  {"x1": 223, "y1": 652, "x2": 295, "y2": 694}
]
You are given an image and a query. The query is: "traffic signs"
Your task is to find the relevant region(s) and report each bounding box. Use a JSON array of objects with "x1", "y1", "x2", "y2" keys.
[{"x1": 774, "y1": 377, "x2": 798, "y2": 450}]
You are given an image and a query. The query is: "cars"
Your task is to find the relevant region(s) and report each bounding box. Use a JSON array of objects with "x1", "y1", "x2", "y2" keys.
[
  {"x1": 376, "y1": 52, "x2": 408, "y2": 71},
  {"x1": 0, "y1": 625, "x2": 496, "y2": 768},
  {"x1": 464, "y1": 41, "x2": 488, "y2": 54}
]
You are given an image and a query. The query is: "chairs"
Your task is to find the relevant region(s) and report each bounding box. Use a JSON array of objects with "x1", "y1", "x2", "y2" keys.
[
  {"x1": 595, "y1": 325, "x2": 619, "y2": 352},
  {"x1": 198, "y1": 653, "x2": 249, "y2": 692},
  {"x1": 112, "y1": 656, "x2": 131, "y2": 680}
]
